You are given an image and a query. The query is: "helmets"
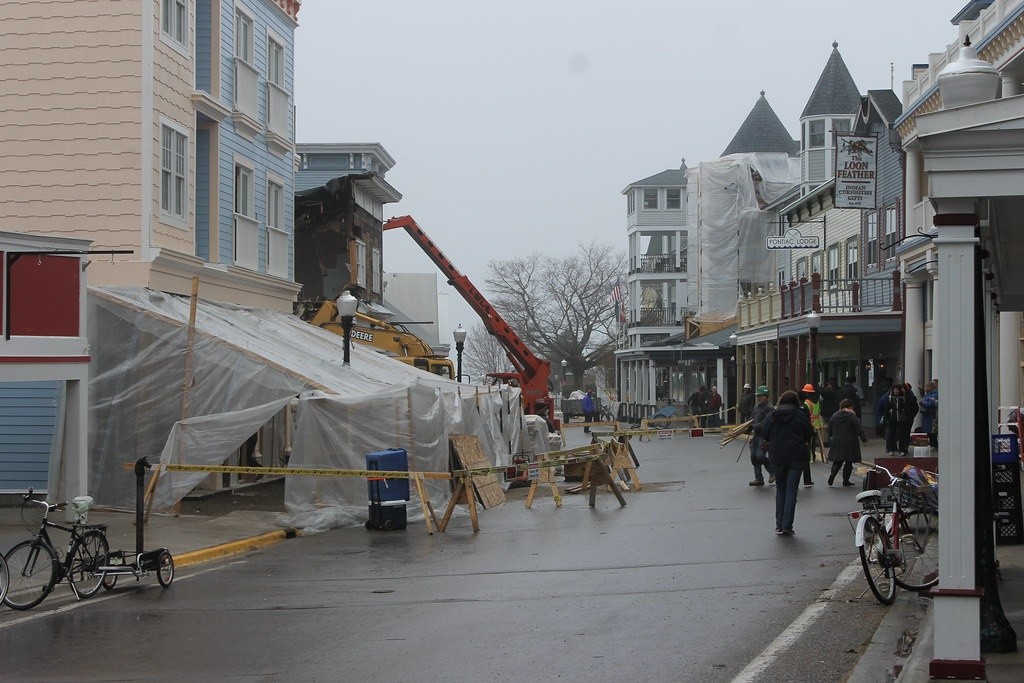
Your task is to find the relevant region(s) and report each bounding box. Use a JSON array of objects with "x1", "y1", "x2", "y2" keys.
[
  {"x1": 802, "y1": 383, "x2": 815, "y2": 393},
  {"x1": 743, "y1": 384, "x2": 751, "y2": 389},
  {"x1": 754, "y1": 385, "x2": 769, "y2": 395}
]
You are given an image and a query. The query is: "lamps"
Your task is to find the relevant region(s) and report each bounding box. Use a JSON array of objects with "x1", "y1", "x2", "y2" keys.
[{"x1": 936, "y1": 33, "x2": 999, "y2": 109}]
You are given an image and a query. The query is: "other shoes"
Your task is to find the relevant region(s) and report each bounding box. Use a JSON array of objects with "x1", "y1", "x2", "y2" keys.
[
  {"x1": 769, "y1": 472, "x2": 776, "y2": 482},
  {"x1": 843, "y1": 480, "x2": 855, "y2": 486},
  {"x1": 890, "y1": 451, "x2": 894, "y2": 456},
  {"x1": 828, "y1": 473, "x2": 834, "y2": 486},
  {"x1": 803, "y1": 481, "x2": 814, "y2": 485},
  {"x1": 899, "y1": 452, "x2": 906, "y2": 456},
  {"x1": 775, "y1": 528, "x2": 795, "y2": 535},
  {"x1": 749, "y1": 478, "x2": 764, "y2": 486}
]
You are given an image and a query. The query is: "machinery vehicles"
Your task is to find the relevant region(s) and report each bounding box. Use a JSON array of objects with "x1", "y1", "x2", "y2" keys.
[
  {"x1": 384, "y1": 215, "x2": 561, "y2": 433},
  {"x1": 561, "y1": 384, "x2": 601, "y2": 423},
  {"x1": 293, "y1": 296, "x2": 455, "y2": 380}
]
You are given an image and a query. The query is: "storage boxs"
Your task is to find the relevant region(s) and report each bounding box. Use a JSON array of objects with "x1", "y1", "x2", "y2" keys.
[
  {"x1": 991, "y1": 434, "x2": 1024, "y2": 545},
  {"x1": 366, "y1": 448, "x2": 409, "y2": 502},
  {"x1": 366, "y1": 501, "x2": 406, "y2": 529}
]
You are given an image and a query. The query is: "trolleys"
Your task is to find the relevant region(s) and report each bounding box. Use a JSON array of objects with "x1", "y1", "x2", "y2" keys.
[
  {"x1": 648, "y1": 401, "x2": 687, "y2": 430},
  {"x1": 365, "y1": 460, "x2": 407, "y2": 531}
]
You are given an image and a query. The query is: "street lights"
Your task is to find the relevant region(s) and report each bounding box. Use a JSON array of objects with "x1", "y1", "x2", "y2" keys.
[
  {"x1": 554, "y1": 374, "x2": 559, "y2": 406},
  {"x1": 806, "y1": 310, "x2": 820, "y2": 388},
  {"x1": 728, "y1": 331, "x2": 737, "y2": 423},
  {"x1": 336, "y1": 290, "x2": 358, "y2": 362},
  {"x1": 453, "y1": 323, "x2": 467, "y2": 382},
  {"x1": 510, "y1": 363, "x2": 515, "y2": 373},
  {"x1": 561, "y1": 358, "x2": 567, "y2": 381}
]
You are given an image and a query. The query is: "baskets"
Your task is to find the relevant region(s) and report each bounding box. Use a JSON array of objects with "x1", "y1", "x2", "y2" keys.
[{"x1": 892, "y1": 471, "x2": 939, "y2": 512}]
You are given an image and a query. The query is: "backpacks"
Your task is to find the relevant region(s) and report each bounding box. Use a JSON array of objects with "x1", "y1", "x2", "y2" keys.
[{"x1": 901, "y1": 464, "x2": 939, "y2": 509}]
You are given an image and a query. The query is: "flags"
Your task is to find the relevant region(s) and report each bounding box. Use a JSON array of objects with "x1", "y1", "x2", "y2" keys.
[{"x1": 608, "y1": 282, "x2": 627, "y2": 323}]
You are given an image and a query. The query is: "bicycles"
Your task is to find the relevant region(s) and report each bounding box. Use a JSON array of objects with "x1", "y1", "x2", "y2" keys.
[
  {"x1": 0, "y1": 487, "x2": 174, "y2": 610},
  {"x1": 0, "y1": 552, "x2": 10, "y2": 605},
  {"x1": 847, "y1": 461, "x2": 939, "y2": 605},
  {"x1": 599, "y1": 404, "x2": 615, "y2": 421}
]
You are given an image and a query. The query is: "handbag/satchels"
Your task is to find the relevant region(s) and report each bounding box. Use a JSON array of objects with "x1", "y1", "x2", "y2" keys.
[
  {"x1": 897, "y1": 409, "x2": 908, "y2": 423},
  {"x1": 919, "y1": 408, "x2": 929, "y2": 415},
  {"x1": 932, "y1": 401, "x2": 939, "y2": 434},
  {"x1": 879, "y1": 416, "x2": 886, "y2": 425}
]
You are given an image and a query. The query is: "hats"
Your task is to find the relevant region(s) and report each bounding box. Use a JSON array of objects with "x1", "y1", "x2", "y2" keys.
[
  {"x1": 903, "y1": 382, "x2": 911, "y2": 393},
  {"x1": 826, "y1": 380, "x2": 835, "y2": 386}
]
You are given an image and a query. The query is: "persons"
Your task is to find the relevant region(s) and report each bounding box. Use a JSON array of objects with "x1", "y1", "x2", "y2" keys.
[
  {"x1": 743, "y1": 386, "x2": 776, "y2": 486},
  {"x1": 737, "y1": 383, "x2": 755, "y2": 423},
  {"x1": 582, "y1": 390, "x2": 595, "y2": 432},
  {"x1": 797, "y1": 375, "x2": 938, "y2": 487},
  {"x1": 762, "y1": 391, "x2": 814, "y2": 535},
  {"x1": 687, "y1": 385, "x2": 723, "y2": 427}
]
